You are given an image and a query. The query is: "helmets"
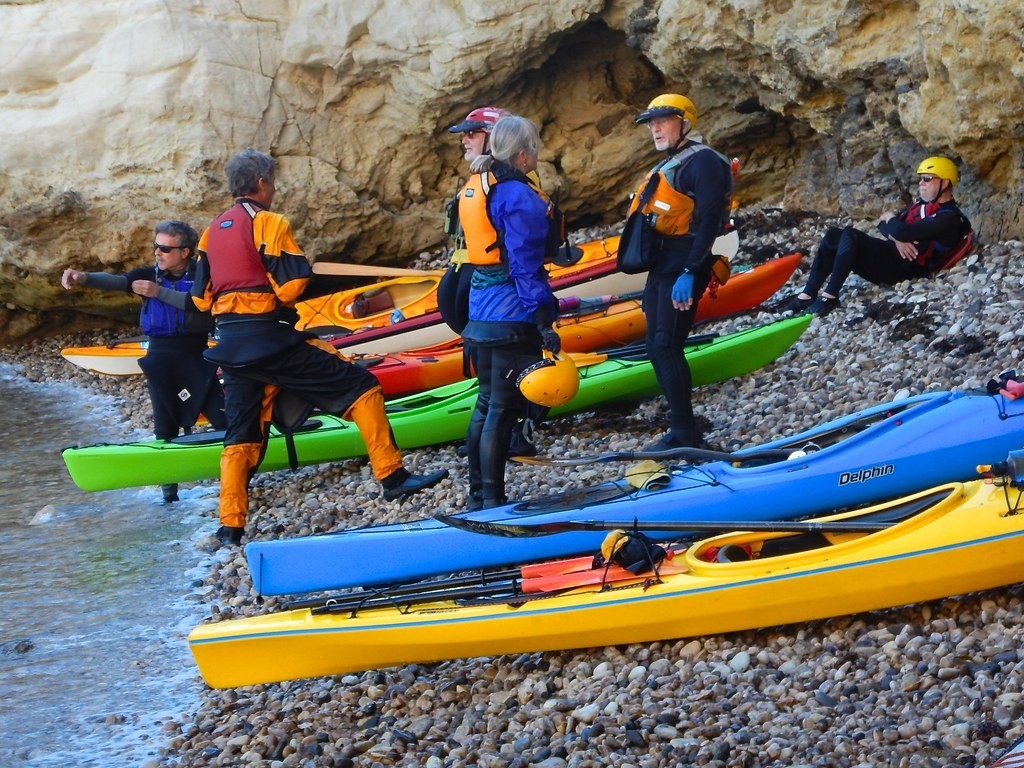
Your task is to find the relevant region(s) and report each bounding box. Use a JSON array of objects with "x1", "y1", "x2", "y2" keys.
[
  {"x1": 447, "y1": 107, "x2": 510, "y2": 133},
  {"x1": 634, "y1": 93, "x2": 698, "y2": 130},
  {"x1": 917, "y1": 156, "x2": 958, "y2": 187},
  {"x1": 516, "y1": 348, "x2": 580, "y2": 407}
]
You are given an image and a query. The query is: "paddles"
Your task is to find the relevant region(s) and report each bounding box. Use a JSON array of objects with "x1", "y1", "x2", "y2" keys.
[
  {"x1": 313, "y1": 261, "x2": 446, "y2": 277},
  {"x1": 433, "y1": 511, "x2": 896, "y2": 536},
  {"x1": 565, "y1": 335, "x2": 720, "y2": 366},
  {"x1": 509, "y1": 446, "x2": 803, "y2": 467},
  {"x1": 285, "y1": 553, "x2": 689, "y2": 613}
]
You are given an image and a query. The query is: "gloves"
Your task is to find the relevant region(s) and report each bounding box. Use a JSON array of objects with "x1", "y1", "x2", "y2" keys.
[{"x1": 540, "y1": 325, "x2": 561, "y2": 354}]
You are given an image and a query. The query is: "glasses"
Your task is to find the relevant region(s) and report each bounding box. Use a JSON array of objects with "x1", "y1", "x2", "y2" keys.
[
  {"x1": 648, "y1": 115, "x2": 680, "y2": 129},
  {"x1": 918, "y1": 174, "x2": 942, "y2": 182},
  {"x1": 463, "y1": 129, "x2": 486, "y2": 139},
  {"x1": 153, "y1": 242, "x2": 187, "y2": 253}
]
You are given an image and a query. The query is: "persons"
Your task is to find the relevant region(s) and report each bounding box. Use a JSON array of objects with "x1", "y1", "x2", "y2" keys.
[
  {"x1": 627, "y1": 93, "x2": 735, "y2": 452},
  {"x1": 62, "y1": 219, "x2": 225, "y2": 442},
  {"x1": 190, "y1": 149, "x2": 450, "y2": 548},
  {"x1": 776, "y1": 156, "x2": 974, "y2": 319},
  {"x1": 458, "y1": 115, "x2": 562, "y2": 512},
  {"x1": 449, "y1": 107, "x2": 541, "y2": 460}
]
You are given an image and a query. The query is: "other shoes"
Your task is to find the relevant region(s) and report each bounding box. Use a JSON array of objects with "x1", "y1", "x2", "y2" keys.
[
  {"x1": 164, "y1": 494, "x2": 179, "y2": 503},
  {"x1": 382, "y1": 466, "x2": 450, "y2": 502},
  {"x1": 771, "y1": 295, "x2": 815, "y2": 313},
  {"x1": 798, "y1": 296, "x2": 841, "y2": 316},
  {"x1": 467, "y1": 495, "x2": 515, "y2": 511},
  {"x1": 458, "y1": 425, "x2": 536, "y2": 462},
  {"x1": 642, "y1": 432, "x2": 709, "y2": 460},
  {"x1": 216, "y1": 526, "x2": 245, "y2": 548}
]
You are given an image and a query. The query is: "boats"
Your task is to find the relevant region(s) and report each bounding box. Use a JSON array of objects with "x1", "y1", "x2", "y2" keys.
[
  {"x1": 356, "y1": 247, "x2": 810, "y2": 398},
  {"x1": 55, "y1": 308, "x2": 819, "y2": 495},
  {"x1": 237, "y1": 363, "x2": 1024, "y2": 605},
  {"x1": 209, "y1": 210, "x2": 747, "y2": 376},
  {"x1": 60, "y1": 156, "x2": 743, "y2": 375},
  {"x1": 187, "y1": 443, "x2": 1024, "y2": 693}
]
